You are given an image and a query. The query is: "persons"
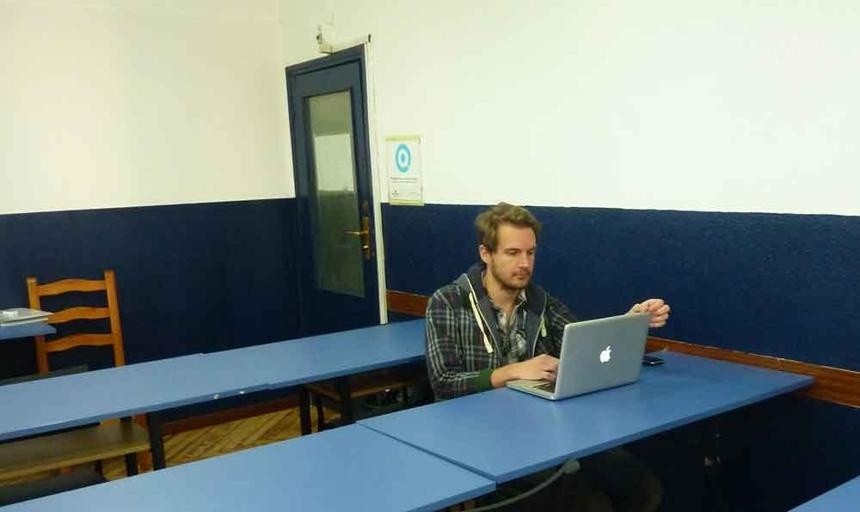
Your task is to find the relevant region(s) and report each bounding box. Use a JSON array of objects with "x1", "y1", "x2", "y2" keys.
[{"x1": 424, "y1": 201, "x2": 671, "y2": 508}]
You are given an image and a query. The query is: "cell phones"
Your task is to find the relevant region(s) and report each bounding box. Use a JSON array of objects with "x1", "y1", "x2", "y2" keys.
[{"x1": 643, "y1": 357, "x2": 665, "y2": 367}]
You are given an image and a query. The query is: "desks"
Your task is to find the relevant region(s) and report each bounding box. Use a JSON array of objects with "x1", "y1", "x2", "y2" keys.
[{"x1": 0, "y1": 308, "x2": 860, "y2": 512}]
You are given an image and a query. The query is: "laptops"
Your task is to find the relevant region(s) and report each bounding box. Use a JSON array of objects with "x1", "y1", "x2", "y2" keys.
[{"x1": 506, "y1": 310, "x2": 652, "y2": 401}]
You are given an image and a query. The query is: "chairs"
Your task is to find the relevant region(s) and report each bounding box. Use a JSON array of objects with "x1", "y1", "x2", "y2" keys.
[{"x1": 24, "y1": 268, "x2": 136, "y2": 477}]
